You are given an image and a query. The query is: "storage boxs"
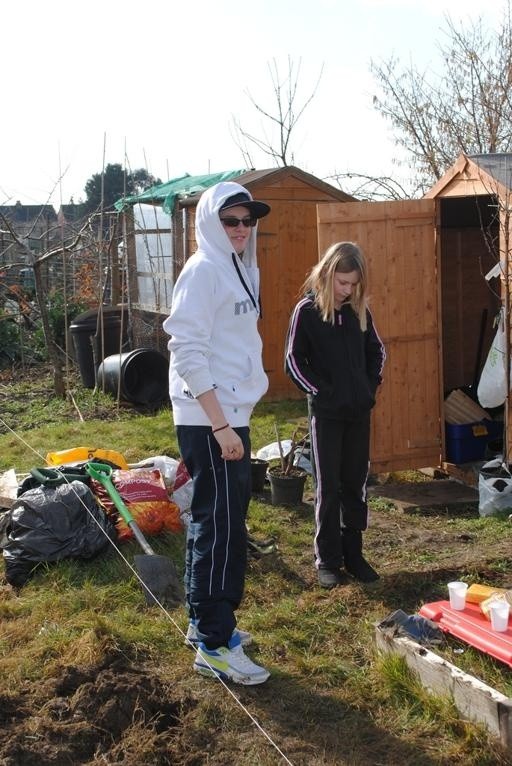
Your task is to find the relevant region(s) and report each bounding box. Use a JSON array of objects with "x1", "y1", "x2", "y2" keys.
[{"x1": 445, "y1": 418, "x2": 505, "y2": 464}]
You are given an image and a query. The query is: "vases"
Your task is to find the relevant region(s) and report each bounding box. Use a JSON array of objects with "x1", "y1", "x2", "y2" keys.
[{"x1": 250, "y1": 458, "x2": 309, "y2": 507}]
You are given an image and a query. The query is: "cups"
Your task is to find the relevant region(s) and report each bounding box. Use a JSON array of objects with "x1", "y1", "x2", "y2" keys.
[
  {"x1": 447, "y1": 582, "x2": 468, "y2": 611},
  {"x1": 487, "y1": 602, "x2": 511, "y2": 632}
]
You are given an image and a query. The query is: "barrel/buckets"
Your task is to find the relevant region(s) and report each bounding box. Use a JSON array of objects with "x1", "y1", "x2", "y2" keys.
[
  {"x1": 69, "y1": 315, "x2": 123, "y2": 388},
  {"x1": 97, "y1": 348, "x2": 169, "y2": 409}
]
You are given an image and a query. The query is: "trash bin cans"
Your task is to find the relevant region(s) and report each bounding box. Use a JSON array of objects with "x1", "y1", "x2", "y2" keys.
[{"x1": 70, "y1": 305, "x2": 169, "y2": 406}]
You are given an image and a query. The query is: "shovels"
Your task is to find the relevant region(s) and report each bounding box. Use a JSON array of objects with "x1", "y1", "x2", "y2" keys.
[{"x1": 82, "y1": 462, "x2": 183, "y2": 612}]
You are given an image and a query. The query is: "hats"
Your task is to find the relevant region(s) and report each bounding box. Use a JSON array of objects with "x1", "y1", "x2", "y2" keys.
[{"x1": 219, "y1": 193, "x2": 270, "y2": 217}]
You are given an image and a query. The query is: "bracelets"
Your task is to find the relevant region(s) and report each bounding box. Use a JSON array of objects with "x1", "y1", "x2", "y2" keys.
[{"x1": 211, "y1": 424, "x2": 230, "y2": 433}]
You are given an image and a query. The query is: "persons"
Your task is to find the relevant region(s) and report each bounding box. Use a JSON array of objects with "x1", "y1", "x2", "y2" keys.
[
  {"x1": 285, "y1": 242, "x2": 386, "y2": 588},
  {"x1": 163, "y1": 179, "x2": 270, "y2": 687}
]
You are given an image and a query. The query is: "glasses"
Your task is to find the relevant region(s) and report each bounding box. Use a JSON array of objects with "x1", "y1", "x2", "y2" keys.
[{"x1": 220, "y1": 217, "x2": 257, "y2": 227}]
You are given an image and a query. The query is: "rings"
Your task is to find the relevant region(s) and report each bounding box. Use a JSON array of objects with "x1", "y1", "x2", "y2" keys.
[{"x1": 230, "y1": 450, "x2": 235, "y2": 454}]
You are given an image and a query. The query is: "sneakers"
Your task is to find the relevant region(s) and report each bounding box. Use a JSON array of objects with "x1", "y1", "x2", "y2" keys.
[
  {"x1": 193, "y1": 633, "x2": 272, "y2": 685},
  {"x1": 344, "y1": 553, "x2": 379, "y2": 583},
  {"x1": 317, "y1": 559, "x2": 345, "y2": 587},
  {"x1": 185, "y1": 618, "x2": 252, "y2": 648}
]
